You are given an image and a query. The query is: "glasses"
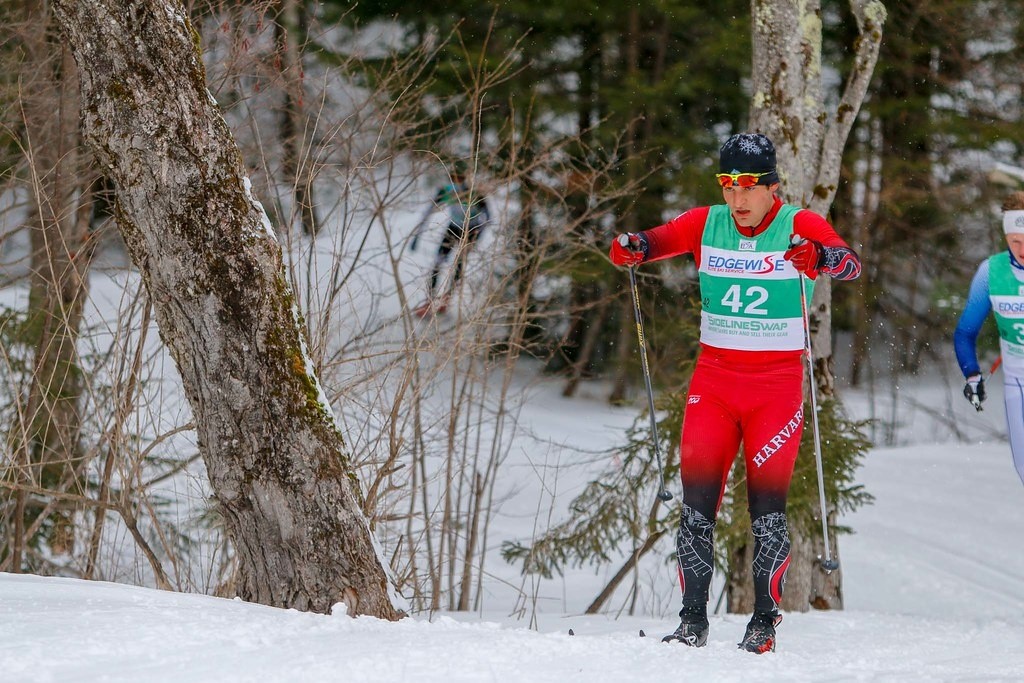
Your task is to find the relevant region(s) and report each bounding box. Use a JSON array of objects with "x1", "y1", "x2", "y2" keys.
[{"x1": 716, "y1": 172, "x2": 771, "y2": 187}]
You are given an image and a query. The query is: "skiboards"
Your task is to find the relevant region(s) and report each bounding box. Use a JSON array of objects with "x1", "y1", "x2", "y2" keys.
[{"x1": 566, "y1": 626, "x2": 648, "y2": 638}]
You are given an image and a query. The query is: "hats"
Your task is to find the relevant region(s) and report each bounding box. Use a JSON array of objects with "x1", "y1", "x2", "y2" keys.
[{"x1": 719, "y1": 132, "x2": 778, "y2": 185}]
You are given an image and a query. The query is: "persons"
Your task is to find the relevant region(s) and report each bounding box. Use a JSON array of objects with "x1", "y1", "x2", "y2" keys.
[
  {"x1": 610, "y1": 133, "x2": 861, "y2": 653},
  {"x1": 417, "y1": 161, "x2": 489, "y2": 316},
  {"x1": 954, "y1": 193, "x2": 1024, "y2": 485}
]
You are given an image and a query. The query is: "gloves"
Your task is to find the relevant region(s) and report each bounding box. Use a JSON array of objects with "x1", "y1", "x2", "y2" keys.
[
  {"x1": 963, "y1": 374, "x2": 986, "y2": 407},
  {"x1": 608, "y1": 232, "x2": 644, "y2": 266},
  {"x1": 784, "y1": 234, "x2": 819, "y2": 272}
]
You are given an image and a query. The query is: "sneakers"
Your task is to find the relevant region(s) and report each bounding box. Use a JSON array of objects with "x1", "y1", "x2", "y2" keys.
[
  {"x1": 662, "y1": 608, "x2": 709, "y2": 646},
  {"x1": 739, "y1": 613, "x2": 776, "y2": 655}
]
